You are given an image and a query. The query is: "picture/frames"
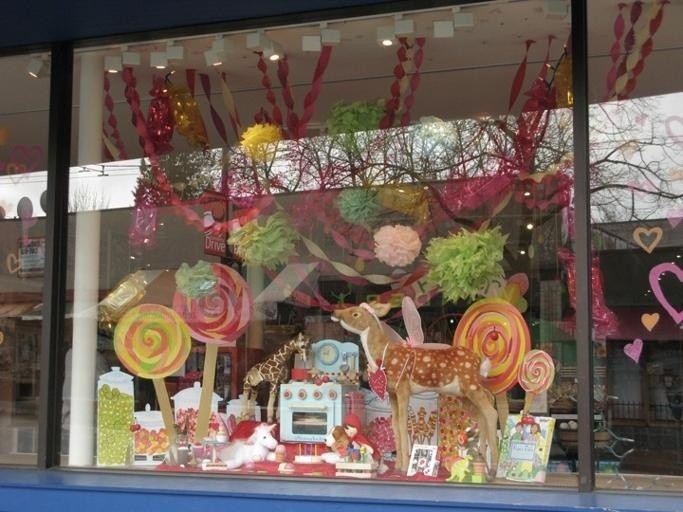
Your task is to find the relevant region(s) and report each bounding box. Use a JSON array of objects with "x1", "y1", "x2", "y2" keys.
[
  {"x1": 406, "y1": 444, "x2": 438, "y2": 476},
  {"x1": 496, "y1": 413, "x2": 556, "y2": 484},
  {"x1": 16, "y1": 236, "x2": 46, "y2": 278}
]
